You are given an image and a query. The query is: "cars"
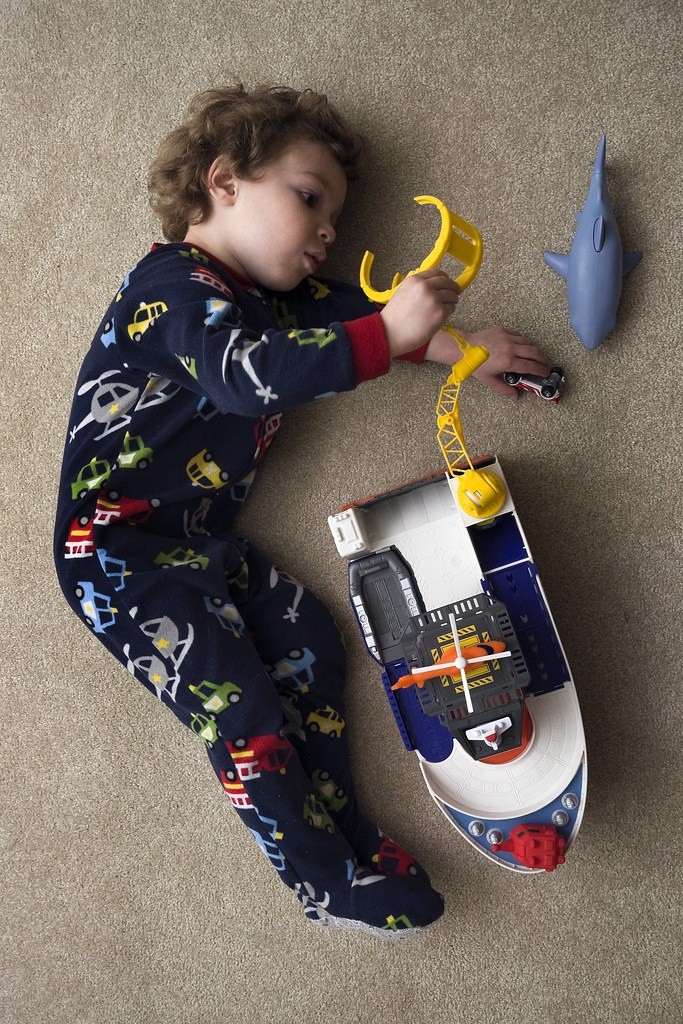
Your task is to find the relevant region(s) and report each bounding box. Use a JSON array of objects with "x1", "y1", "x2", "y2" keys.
[{"x1": 501, "y1": 367, "x2": 566, "y2": 404}]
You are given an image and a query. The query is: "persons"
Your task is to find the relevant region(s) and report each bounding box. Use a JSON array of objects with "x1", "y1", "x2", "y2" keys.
[{"x1": 52, "y1": 83, "x2": 550, "y2": 932}]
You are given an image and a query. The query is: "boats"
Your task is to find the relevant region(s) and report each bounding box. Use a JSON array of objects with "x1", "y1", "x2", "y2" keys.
[{"x1": 325, "y1": 451, "x2": 591, "y2": 879}]
